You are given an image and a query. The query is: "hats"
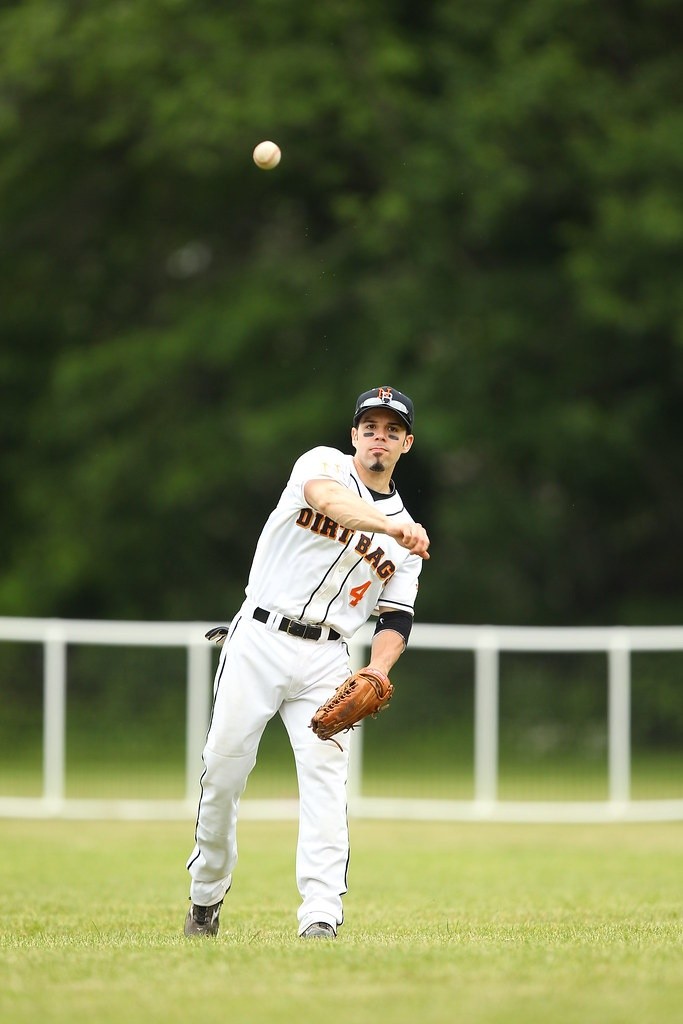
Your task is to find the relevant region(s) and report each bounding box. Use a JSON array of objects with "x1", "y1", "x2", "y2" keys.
[{"x1": 353, "y1": 386, "x2": 413, "y2": 434}]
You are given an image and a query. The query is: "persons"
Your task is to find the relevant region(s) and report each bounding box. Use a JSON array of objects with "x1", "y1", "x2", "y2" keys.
[{"x1": 185, "y1": 385, "x2": 432, "y2": 943}]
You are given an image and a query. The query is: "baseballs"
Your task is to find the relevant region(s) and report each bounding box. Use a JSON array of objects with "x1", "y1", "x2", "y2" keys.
[{"x1": 253, "y1": 139, "x2": 284, "y2": 171}]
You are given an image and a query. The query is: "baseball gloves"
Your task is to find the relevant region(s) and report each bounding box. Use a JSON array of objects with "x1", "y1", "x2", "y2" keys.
[{"x1": 311, "y1": 673, "x2": 392, "y2": 741}]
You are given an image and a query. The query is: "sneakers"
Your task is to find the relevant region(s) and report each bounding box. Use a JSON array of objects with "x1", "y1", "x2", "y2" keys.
[
  {"x1": 299, "y1": 922, "x2": 335, "y2": 940},
  {"x1": 184, "y1": 881, "x2": 231, "y2": 942}
]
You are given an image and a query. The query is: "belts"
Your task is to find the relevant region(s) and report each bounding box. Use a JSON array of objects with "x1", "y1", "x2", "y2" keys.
[{"x1": 252, "y1": 607, "x2": 340, "y2": 641}]
